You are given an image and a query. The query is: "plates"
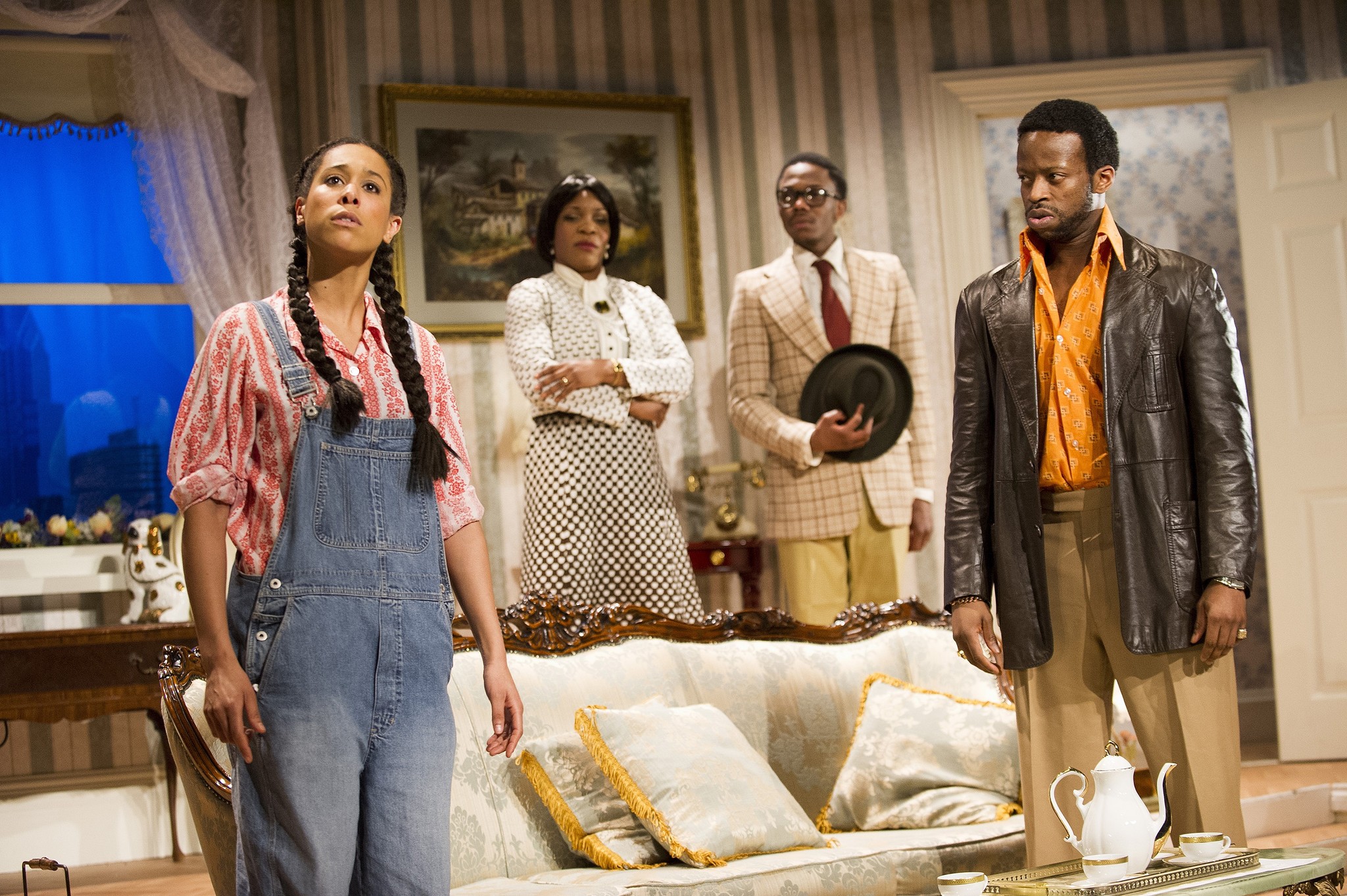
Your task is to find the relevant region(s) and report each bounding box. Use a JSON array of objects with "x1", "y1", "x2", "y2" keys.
[
  {"x1": 1066, "y1": 874, "x2": 1148, "y2": 886},
  {"x1": 1162, "y1": 852, "x2": 1243, "y2": 868},
  {"x1": 1147, "y1": 850, "x2": 1181, "y2": 868}
]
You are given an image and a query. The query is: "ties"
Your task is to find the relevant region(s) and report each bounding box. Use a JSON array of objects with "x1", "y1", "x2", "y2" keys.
[{"x1": 811, "y1": 260, "x2": 852, "y2": 351}]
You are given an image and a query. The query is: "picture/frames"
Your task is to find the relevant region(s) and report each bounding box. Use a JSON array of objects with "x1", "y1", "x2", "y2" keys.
[{"x1": 378, "y1": 80, "x2": 707, "y2": 342}]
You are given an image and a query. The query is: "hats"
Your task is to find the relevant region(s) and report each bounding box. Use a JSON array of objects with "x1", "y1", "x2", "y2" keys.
[{"x1": 800, "y1": 343, "x2": 914, "y2": 462}]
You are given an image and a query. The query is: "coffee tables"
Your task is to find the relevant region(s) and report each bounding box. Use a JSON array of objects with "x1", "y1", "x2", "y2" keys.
[{"x1": 924, "y1": 845, "x2": 1347, "y2": 896}]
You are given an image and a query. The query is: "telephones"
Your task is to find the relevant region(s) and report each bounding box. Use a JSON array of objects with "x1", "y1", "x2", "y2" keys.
[{"x1": 685, "y1": 457, "x2": 770, "y2": 538}]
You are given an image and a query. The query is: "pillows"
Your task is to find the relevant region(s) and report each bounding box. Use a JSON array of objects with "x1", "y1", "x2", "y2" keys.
[
  {"x1": 570, "y1": 704, "x2": 835, "y2": 867},
  {"x1": 514, "y1": 732, "x2": 676, "y2": 870},
  {"x1": 814, "y1": 672, "x2": 1024, "y2": 833}
]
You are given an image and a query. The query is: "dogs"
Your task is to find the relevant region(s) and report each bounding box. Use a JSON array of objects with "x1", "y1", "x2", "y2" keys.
[{"x1": 120, "y1": 519, "x2": 191, "y2": 626}]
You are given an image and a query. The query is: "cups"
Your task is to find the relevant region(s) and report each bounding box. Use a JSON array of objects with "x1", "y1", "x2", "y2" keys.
[
  {"x1": 1082, "y1": 854, "x2": 1129, "y2": 884},
  {"x1": 937, "y1": 871, "x2": 988, "y2": 896},
  {"x1": 1179, "y1": 832, "x2": 1231, "y2": 861}
]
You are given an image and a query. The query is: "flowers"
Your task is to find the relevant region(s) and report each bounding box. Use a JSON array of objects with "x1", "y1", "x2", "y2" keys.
[{"x1": 0, "y1": 492, "x2": 122, "y2": 550}]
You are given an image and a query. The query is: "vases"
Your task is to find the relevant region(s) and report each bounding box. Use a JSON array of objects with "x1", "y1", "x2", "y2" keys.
[{"x1": 0, "y1": 542, "x2": 128, "y2": 597}]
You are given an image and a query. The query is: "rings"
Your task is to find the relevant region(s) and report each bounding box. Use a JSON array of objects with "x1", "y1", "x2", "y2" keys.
[
  {"x1": 562, "y1": 377, "x2": 569, "y2": 385},
  {"x1": 1237, "y1": 629, "x2": 1247, "y2": 639},
  {"x1": 957, "y1": 650, "x2": 967, "y2": 659}
]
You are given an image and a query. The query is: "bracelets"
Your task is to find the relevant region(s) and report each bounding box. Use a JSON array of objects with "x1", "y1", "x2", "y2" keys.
[
  {"x1": 1214, "y1": 577, "x2": 1245, "y2": 590},
  {"x1": 609, "y1": 357, "x2": 623, "y2": 387},
  {"x1": 950, "y1": 596, "x2": 983, "y2": 605}
]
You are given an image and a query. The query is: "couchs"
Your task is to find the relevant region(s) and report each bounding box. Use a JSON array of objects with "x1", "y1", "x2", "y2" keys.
[{"x1": 156, "y1": 589, "x2": 1162, "y2": 896}]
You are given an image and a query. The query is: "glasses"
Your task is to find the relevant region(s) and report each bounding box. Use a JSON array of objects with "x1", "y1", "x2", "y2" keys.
[{"x1": 776, "y1": 187, "x2": 844, "y2": 207}]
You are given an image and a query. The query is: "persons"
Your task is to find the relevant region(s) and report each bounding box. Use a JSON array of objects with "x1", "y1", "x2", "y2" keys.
[
  {"x1": 727, "y1": 152, "x2": 936, "y2": 625},
  {"x1": 504, "y1": 172, "x2": 703, "y2": 624},
  {"x1": 164, "y1": 140, "x2": 522, "y2": 896},
  {"x1": 944, "y1": 98, "x2": 1258, "y2": 896}
]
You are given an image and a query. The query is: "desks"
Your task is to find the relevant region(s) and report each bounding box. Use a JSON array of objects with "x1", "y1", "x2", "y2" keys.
[
  {"x1": 0, "y1": 618, "x2": 199, "y2": 864},
  {"x1": 681, "y1": 515, "x2": 761, "y2": 612}
]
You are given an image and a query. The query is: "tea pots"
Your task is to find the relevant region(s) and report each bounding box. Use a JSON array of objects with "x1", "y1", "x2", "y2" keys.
[{"x1": 1048, "y1": 740, "x2": 1178, "y2": 875}]
